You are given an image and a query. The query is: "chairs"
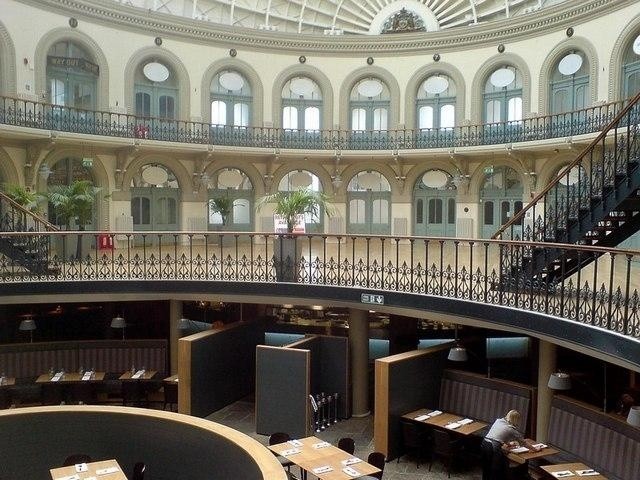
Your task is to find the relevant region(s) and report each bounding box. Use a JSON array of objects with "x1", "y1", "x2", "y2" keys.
[
  {"x1": 338, "y1": 438, "x2": 356, "y2": 455},
  {"x1": 353, "y1": 452, "x2": 385, "y2": 480},
  {"x1": 129, "y1": 461, "x2": 147, "y2": 480},
  {"x1": 62, "y1": 454, "x2": 90, "y2": 466},
  {"x1": 431, "y1": 429, "x2": 466, "y2": 478},
  {"x1": 397, "y1": 423, "x2": 429, "y2": 469},
  {"x1": 269, "y1": 432, "x2": 296, "y2": 472}
]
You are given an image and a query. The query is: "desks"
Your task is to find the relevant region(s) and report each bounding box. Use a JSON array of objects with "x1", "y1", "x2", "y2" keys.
[
  {"x1": 400, "y1": 408, "x2": 489, "y2": 474},
  {"x1": 539, "y1": 463, "x2": 608, "y2": 480},
  {"x1": 267, "y1": 436, "x2": 383, "y2": 480},
  {"x1": 49, "y1": 458, "x2": 129, "y2": 480},
  {"x1": 501, "y1": 438, "x2": 560, "y2": 464},
  {"x1": 0, "y1": 371, "x2": 179, "y2": 412}
]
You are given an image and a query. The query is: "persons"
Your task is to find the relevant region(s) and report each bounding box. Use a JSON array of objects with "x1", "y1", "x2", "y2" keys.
[{"x1": 481, "y1": 410, "x2": 541, "y2": 480}]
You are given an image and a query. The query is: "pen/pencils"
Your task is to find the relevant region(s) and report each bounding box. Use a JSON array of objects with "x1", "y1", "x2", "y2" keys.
[
  {"x1": 317, "y1": 444, "x2": 326, "y2": 446},
  {"x1": 557, "y1": 474, "x2": 568, "y2": 475},
  {"x1": 100, "y1": 469, "x2": 108, "y2": 473},
  {"x1": 292, "y1": 441, "x2": 299, "y2": 446},
  {"x1": 286, "y1": 450, "x2": 296, "y2": 453},
  {"x1": 317, "y1": 468, "x2": 328, "y2": 471},
  {"x1": 583, "y1": 471, "x2": 595, "y2": 473},
  {"x1": 69, "y1": 476, "x2": 76, "y2": 479},
  {"x1": 347, "y1": 460, "x2": 358, "y2": 462},
  {"x1": 347, "y1": 469, "x2": 355, "y2": 474},
  {"x1": 79, "y1": 464, "x2": 82, "y2": 471}
]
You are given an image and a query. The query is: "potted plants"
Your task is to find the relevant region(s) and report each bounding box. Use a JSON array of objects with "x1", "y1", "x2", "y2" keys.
[
  {"x1": 45, "y1": 183, "x2": 103, "y2": 261},
  {"x1": 203, "y1": 192, "x2": 245, "y2": 247},
  {"x1": 255, "y1": 189, "x2": 339, "y2": 282}
]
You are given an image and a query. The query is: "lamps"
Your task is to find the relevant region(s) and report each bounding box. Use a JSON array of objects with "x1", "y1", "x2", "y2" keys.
[
  {"x1": 547, "y1": 345, "x2": 572, "y2": 390},
  {"x1": 110, "y1": 302, "x2": 126, "y2": 328},
  {"x1": 626, "y1": 406, "x2": 640, "y2": 426},
  {"x1": 18, "y1": 314, "x2": 36, "y2": 331},
  {"x1": 447, "y1": 323, "x2": 469, "y2": 361}
]
